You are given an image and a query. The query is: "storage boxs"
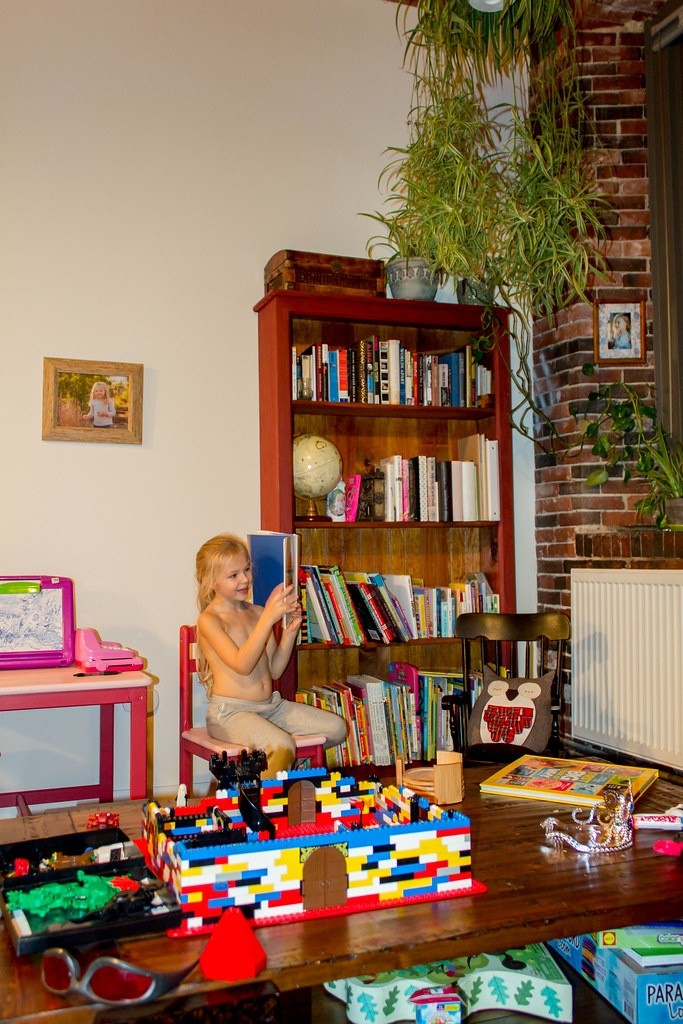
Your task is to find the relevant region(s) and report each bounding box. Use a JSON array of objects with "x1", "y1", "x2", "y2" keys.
[{"x1": 262, "y1": 248, "x2": 388, "y2": 298}]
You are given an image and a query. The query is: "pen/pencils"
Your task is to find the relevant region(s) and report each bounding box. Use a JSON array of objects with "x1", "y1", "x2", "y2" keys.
[{"x1": 73, "y1": 670, "x2": 119, "y2": 677}]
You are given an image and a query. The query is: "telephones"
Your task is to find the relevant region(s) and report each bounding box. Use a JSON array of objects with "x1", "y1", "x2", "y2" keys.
[{"x1": 76, "y1": 628, "x2": 143, "y2": 673}]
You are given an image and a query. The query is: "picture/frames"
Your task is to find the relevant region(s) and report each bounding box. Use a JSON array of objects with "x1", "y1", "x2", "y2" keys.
[
  {"x1": 592, "y1": 295, "x2": 647, "y2": 366},
  {"x1": 42, "y1": 356, "x2": 144, "y2": 446}
]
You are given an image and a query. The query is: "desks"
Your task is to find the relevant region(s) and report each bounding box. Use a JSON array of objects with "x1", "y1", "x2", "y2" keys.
[
  {"x1": 1, "y1": 754, "x2": 683, "y2": 1024},
  {"x1": 0, "y1": 663, "x2": 153, "y2": 819}
]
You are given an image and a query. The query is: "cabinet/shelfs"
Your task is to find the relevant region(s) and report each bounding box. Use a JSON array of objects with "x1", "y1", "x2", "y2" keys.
[{"x1": 252, "y1": 289, "x2": 521, "y2": 773}]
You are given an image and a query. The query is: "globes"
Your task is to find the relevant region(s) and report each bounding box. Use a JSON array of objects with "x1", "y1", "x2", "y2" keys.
[{"x1": 292, "y1": 433, "x2": 343, "y2": 522}]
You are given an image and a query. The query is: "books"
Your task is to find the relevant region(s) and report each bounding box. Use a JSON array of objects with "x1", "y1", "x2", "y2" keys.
[
  {"x1": 479, "y1": 752, "x2": 660, "y2": 810},
  {"x1": 291, "y1": 331, "x2": 505, "y2": 767}
]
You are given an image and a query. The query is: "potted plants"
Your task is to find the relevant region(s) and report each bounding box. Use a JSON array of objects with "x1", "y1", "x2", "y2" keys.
[
  {"x1": 439, "y1": 120, "x2": 505, "y2": 305},
  {"x1": 566, "y1": 363, "x2": 683, "y2": 531},
  {"x1": 356, "y1": 111, "x2": 454, "y2": 302}
]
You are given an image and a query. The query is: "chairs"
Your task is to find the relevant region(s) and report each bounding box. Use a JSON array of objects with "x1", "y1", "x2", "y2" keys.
[
  {"x1": 440, "y1": 612, "x2": 571, "y2": 767},
  {"x1": 180, "y1": 623, "x2": 327, "y2": 800}
]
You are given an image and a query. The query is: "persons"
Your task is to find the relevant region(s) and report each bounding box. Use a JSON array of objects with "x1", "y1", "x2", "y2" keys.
[
  {"x1": 81, "y1": 381, "x2": 116, "y2": 429},
  {"x1": 195, "y1": 532, "x2": 349, "y2": 782},
  {"x1": 612, "y1": 314, "x2": 631, "y2": 349}
]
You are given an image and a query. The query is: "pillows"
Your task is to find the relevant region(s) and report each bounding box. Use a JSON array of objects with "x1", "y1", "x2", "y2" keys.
[{"x1": 464, "y1": 660, "x2": 558, "y2": 763}]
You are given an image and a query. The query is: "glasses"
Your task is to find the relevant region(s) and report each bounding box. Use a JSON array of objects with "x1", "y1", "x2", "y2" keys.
[{"x1": 42, "y1": 937, "x2": 199, "y2": 1005}]
